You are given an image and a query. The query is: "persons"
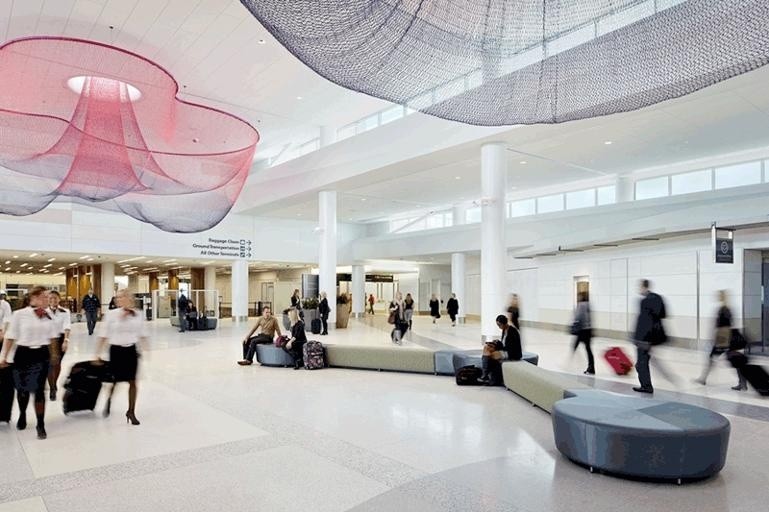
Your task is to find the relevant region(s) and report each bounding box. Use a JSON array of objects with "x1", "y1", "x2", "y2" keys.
[
  {"x1": 477, "y1": 315, "x2": 522, "y2": 385},
  {"x1": 628, "y1": 279, "x2": 680, "y2": 393},
  {"x1": 558, "y1": 292, "x2": 595, "y2": 375},
  {"x1": 280, "y1": 309, "x2": 307, "y2": 369},
  {"x1": 319, "y1": 292, "x2": 330, "y2": 334},
  {"x1": 388, "y1": 292, "x2": 409, "y2": 343},
  {"x1": 0, "y1": 288, "x2": 147, "y2": 439},
  {"x1": 368, "y1": 294, "x2": 374, "y2": 315},
  {"x1": 237, "y1": 306, "x2": 281, "y2": 364},
  {"x1": 507, "y1": 294, "x2": 521, "y2": 332},
  {"x1": 691, "y1": 291, "x2": 748, "y2": 392},
  {"x1": 291, "y1": 290, "x2": 306, "y2": 324},
  {"x1": 404, "y1": 294, "x2": 414, "y2": 330},
  {"x1": 429, "y1": 293, "x2": 440, "y2": 324},
  {"x1": 177, "y1": 289, "x2": 197, "y2": 332},
  {"x1": 446, "y1": 294, "x2": 458, "y2": 327}
]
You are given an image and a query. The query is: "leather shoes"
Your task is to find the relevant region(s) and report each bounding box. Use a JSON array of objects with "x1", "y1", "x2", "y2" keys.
[
  {"x1": 238, "y1": 361, "x2": 251, "y2": 365},
  {"x1": 17, "y1": 413, "x2": 27, "y2": 430},
  {"x1": 37, "y1": 424, "x2": 47, "y2": 439},
  {"x1": 633, "y1": 386, "x2": 653, "y2": 393}
]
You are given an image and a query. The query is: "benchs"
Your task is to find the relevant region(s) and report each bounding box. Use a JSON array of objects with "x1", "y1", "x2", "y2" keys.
[{"x1": 169, "y1": 314, "x2": 218, "y2": 332}]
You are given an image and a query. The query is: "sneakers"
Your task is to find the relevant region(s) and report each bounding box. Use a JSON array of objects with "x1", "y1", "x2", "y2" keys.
[{"x1": 476, "y1": 376, "x2": 490, "y2": 383}]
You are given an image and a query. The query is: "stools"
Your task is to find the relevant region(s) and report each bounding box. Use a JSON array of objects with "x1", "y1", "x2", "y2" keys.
[
  {"x1": 551, "y1": 387, "x2": 730, "y2": 486},
  {"x1": 434, "y1": 347, "x2": 593, "y2": 416},
  {"x1": 324, "y1": 341, "x2": 436, "y2": 374},
  {"x1": 256, "y1": 344, "x2": 295, "y2": 368}
]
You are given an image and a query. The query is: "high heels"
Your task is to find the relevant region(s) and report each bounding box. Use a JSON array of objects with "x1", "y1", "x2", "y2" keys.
[{"x1": 127, "y1": 409, "x2": 139, "y2": 425}]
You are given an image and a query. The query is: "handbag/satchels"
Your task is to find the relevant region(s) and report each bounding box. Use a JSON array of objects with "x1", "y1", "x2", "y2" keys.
[
  {"x1": 276, "y1": 336, "x2": 285, "y2": 346},
  {"x1": 389, "y1": 312, "x2": 395, "y2": 323},
  {"x1": 456, "y1": 365, "x2": 482, "y2": 385},
  {"x1": 728, "y1": 328, "x2": 748, "y2": 368}
]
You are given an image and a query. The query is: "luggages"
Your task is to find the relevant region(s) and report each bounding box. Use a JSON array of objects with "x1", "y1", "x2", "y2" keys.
[
  {"x1": 63, "y1": 361, "x2": 102, "y2": 414},
  {"x1": 303, "y1": 341, "x2": 324, "y2": 369},
  {"x1": 740, "y1": 364, "x2": 769, "y2": 396},
  {"x1": 311, "y1": 312, "x2": 321, "y2": 333},
  {"x1": 605, "y1": 347, "x2": 632, "y2": 375}
]
